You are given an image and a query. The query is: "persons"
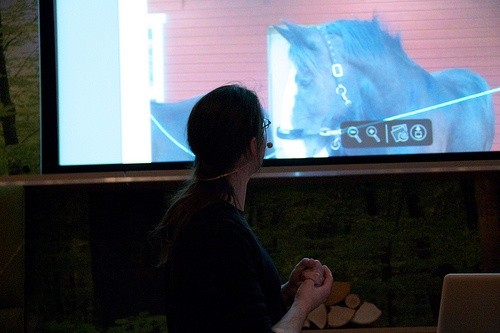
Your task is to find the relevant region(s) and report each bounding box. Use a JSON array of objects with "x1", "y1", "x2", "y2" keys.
[{"x1": 147, "y1": 84, "x2": 334, "y2": 333}]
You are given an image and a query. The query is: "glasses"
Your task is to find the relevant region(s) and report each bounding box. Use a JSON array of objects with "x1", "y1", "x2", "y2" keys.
[{"x1": 261, "y1": 118, "x2": 271, "y2": 129}]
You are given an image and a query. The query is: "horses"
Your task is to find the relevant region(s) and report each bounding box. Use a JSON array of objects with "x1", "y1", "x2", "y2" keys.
[{"x1": 273, "y1": 16, "x2": 495, "y2": 156}]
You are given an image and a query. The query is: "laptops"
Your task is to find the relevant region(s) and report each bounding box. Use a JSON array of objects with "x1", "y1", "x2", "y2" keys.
[{"x1": 436, "y1": 274, "x2": 500, "y2": 333}]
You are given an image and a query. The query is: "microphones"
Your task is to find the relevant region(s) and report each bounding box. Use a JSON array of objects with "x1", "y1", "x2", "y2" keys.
[{"x1": 267, "y1": 142, "x2": 273, "y2": 148}]
